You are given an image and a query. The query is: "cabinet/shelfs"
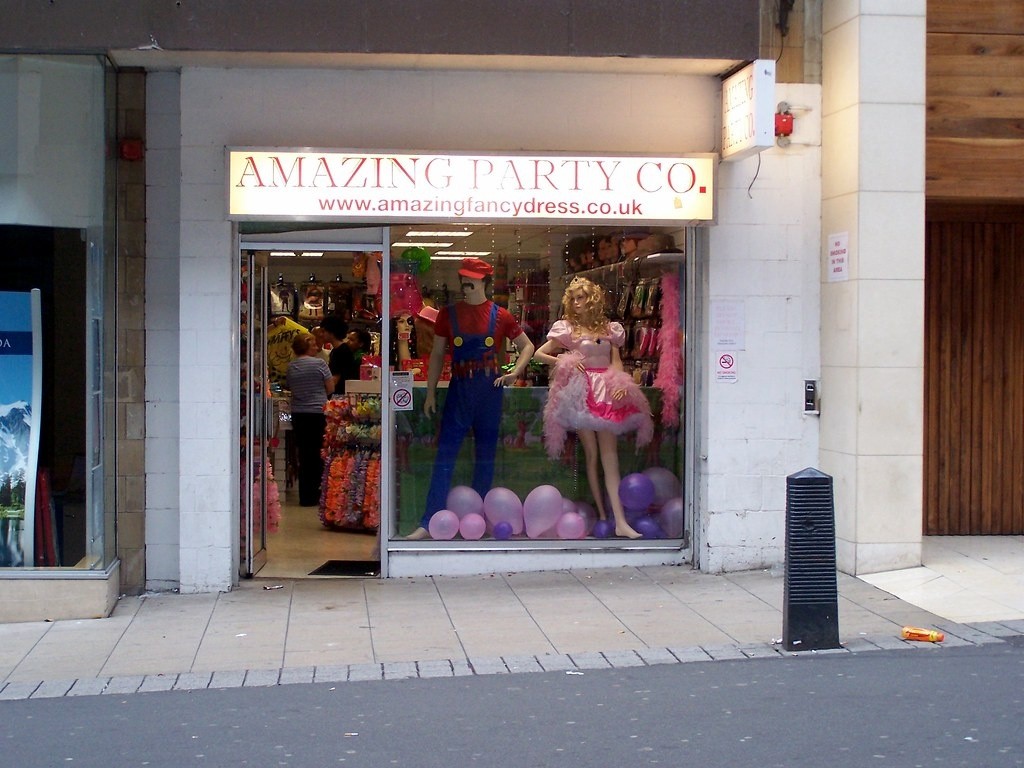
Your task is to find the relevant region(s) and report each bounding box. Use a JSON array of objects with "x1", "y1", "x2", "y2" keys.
[{"x1": 554, "y1": 261, "x2": 662, "y2": 387}]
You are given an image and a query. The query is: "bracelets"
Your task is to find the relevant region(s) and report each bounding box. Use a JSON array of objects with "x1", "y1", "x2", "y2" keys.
[{"x1": 273, "y1": 320, "x2": 277, "y2": 327}]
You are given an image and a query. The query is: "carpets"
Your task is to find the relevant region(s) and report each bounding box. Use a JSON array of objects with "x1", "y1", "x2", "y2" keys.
[{"x1": 307, "y1": 560, "x2": 380, "y2": 576}]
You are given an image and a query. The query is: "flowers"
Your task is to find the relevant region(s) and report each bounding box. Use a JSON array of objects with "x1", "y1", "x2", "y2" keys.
[
  {"x1": 319, "y1": 395, "x2": 379, "y2": 527},
  {"x1": 254, "y1": 457, "x2": 281, "y2": 536}
]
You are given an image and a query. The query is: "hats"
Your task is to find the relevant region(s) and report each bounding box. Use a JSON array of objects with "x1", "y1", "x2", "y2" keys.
[{"x1": 458, "y1": 258, "x2": 494, "y2": 279}]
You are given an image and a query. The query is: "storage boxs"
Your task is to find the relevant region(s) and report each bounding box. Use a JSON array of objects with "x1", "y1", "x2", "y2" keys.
[{"x1": 504, "y1": 259, "x2": 540, "y2": 284}]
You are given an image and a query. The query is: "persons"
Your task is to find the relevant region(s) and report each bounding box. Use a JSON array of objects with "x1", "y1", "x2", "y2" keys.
[
  {"x1": 320, "y1": 315, "x2": 359, "y2": 400},
  {"x1": 310, "y1": 325, "x2": 329, "y2": 362},
  {"x1": 563, "y1": 231, "x2": 649, "y2": 271},
  {"x1": 389, "y1": 313, "x2": 417, "y2": 372},
  {"x1": 347, "y1": 328, "x2": 371, "y2": 366},
  {"x1": 268, "y1": 317, "x2": 286, "y2": 332},
  {"x1": 406, "y1": 260, "x2": 534, "y2": 540},
  {"x1": 285, "y1": 333, "x2": 335, "y2": 507},
  {"x1": 534, "y1": 275, "x2": 654, "y2": 539}
]
